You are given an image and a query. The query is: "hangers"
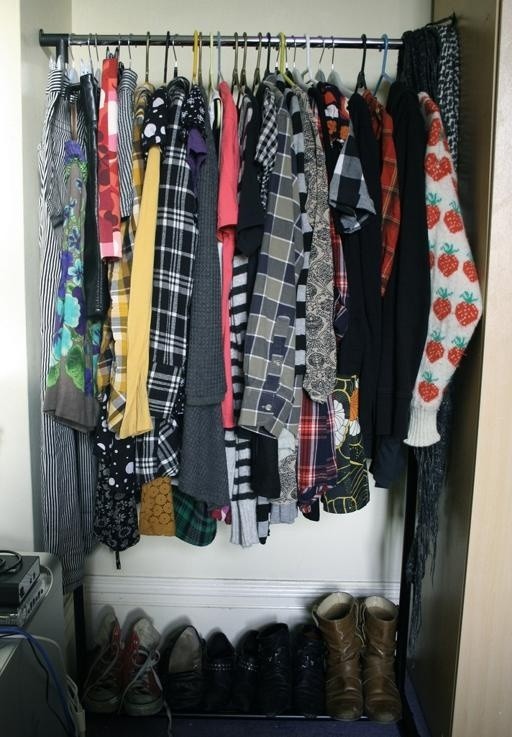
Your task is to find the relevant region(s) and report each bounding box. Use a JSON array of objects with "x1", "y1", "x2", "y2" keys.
[{"x1": 48, "y1": 32, "x2": 423, "y2": 100}]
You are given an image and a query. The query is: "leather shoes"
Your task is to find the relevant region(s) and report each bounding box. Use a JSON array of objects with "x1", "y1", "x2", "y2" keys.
[
  {"x1": 206, "y1": 633, "x2": 236, "y2": 710},
  {"x1": 296, "y1": 625, "x2": 324, "y2": 720},
  {"x1": 259, "y1": 625, "x2": 290, "y2": 714},
  {"x1": 166, "y1": 626, "x2": 203, "y2": 712},
  {"x1": 240, "y1": 632, "x2": 263, "y2": 710}
]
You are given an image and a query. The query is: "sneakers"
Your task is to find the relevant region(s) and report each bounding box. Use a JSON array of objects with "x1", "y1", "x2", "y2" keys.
[
  {"x1": 85, "y1": 614, "x2": 124, "y2": 713},
  {"x1": 125, "y1": 619, "x2": 165, "y2": 717}
]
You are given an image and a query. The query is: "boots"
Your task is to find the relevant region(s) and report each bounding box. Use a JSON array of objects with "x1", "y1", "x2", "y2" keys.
[
  {"x1": 312, "y1": 592, "x2": 364, "y2": 721},
  {"x1": 363, "y1": 596, "x2": 402, "y2": 723}
]
